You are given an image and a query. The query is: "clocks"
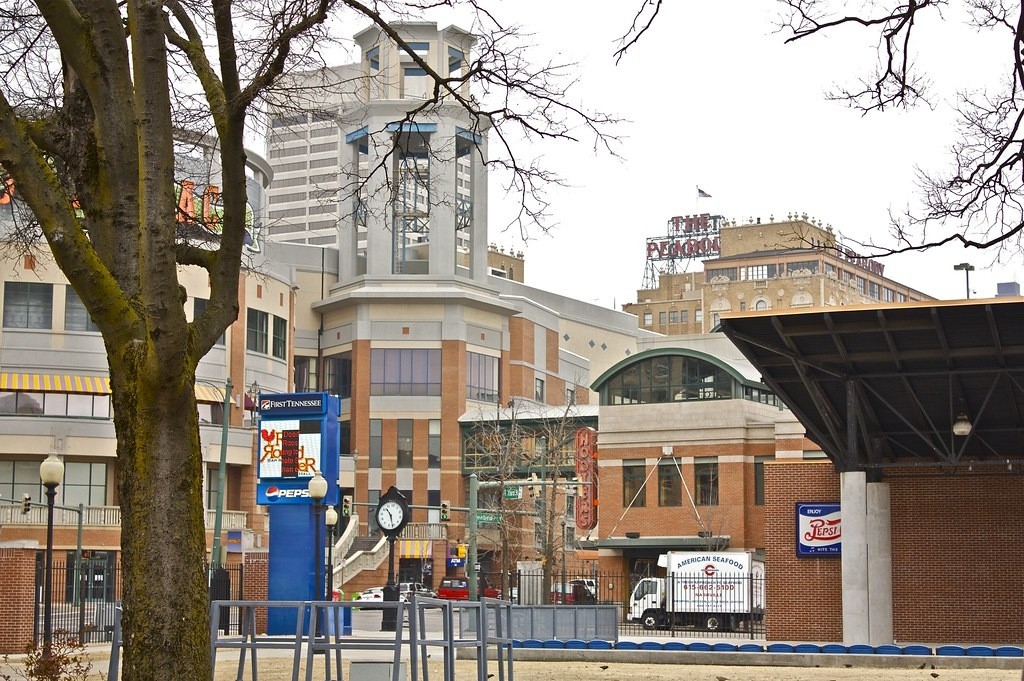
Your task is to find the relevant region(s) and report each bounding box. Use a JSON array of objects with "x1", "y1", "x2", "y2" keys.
[{"x1": 377, "y1": 501, "x2": 404, "y2": 530}]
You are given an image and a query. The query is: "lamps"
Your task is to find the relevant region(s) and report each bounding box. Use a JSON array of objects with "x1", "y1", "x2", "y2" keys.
[
  {"x1": 697, "y1": 531, "x2": 713, "y2": 537},
  {"x1": 953, "y1": 398, "x2": 973, "y2": 436},
  {"x1": 626, "y1": 532, "x2": 640, "y2": 538}
]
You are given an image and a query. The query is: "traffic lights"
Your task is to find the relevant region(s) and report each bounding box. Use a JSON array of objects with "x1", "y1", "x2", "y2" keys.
[
  {"x1": 525, "y1": 473, "x2": 540, "y2": 499},
  {"x1": 81, "y1": 549, "x2": 96, "y2": 559},
  {"x1": 449, "y1": 543, "x2": 466, "y2": 558},
  {"x1": 341, "y1": 494, "x2": 352, "y2": 517},
  {"x1": 440, "y1": 500, "x2": 450, "y2": 520},
  {"x1": 20, "y1": 493, "x2": 32, "y2": 516}
]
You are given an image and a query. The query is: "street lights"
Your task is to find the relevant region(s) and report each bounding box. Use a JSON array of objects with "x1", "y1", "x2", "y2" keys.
[
  {"x1": 559, "y1": 515, "x2": 567, "y2": 605},
  {"x1": 326, "y1": 505, "x2": 338, "y2": 601},
  {"x1": 33, "y1": 449, "x2": 65, "y2": 676},
  {"x1": 308, "y1": 470, "x2": 325, "y2": 654},
  {"x1": 953, "y1": 262, "x2": 975, "y2": 299}
]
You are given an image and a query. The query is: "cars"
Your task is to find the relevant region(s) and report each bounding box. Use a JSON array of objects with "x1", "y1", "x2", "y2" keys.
[{"x1": 356, "y1": 586, "x2": 410, "y2": 610}]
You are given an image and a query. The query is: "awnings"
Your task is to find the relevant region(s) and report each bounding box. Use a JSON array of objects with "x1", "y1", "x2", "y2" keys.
[{"x1": 400, "y1": 541, "x2": 432, "y2": 558}]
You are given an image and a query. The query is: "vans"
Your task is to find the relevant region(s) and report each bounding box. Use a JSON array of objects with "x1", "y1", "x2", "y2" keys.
[
  {"x1": 552, "y1": 577, "x2": 599, "y2": 605},
  {"x1": 395, "y1": 581, "x2": 439, "y2": 608},
  {"x1": 436, "y1": 575, "x2": 502, "y2": 601}
]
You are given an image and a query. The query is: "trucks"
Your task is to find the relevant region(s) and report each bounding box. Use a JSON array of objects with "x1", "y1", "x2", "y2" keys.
[{"x1": 625, "y1": 550, "x2": 766, "y2": 631}]
